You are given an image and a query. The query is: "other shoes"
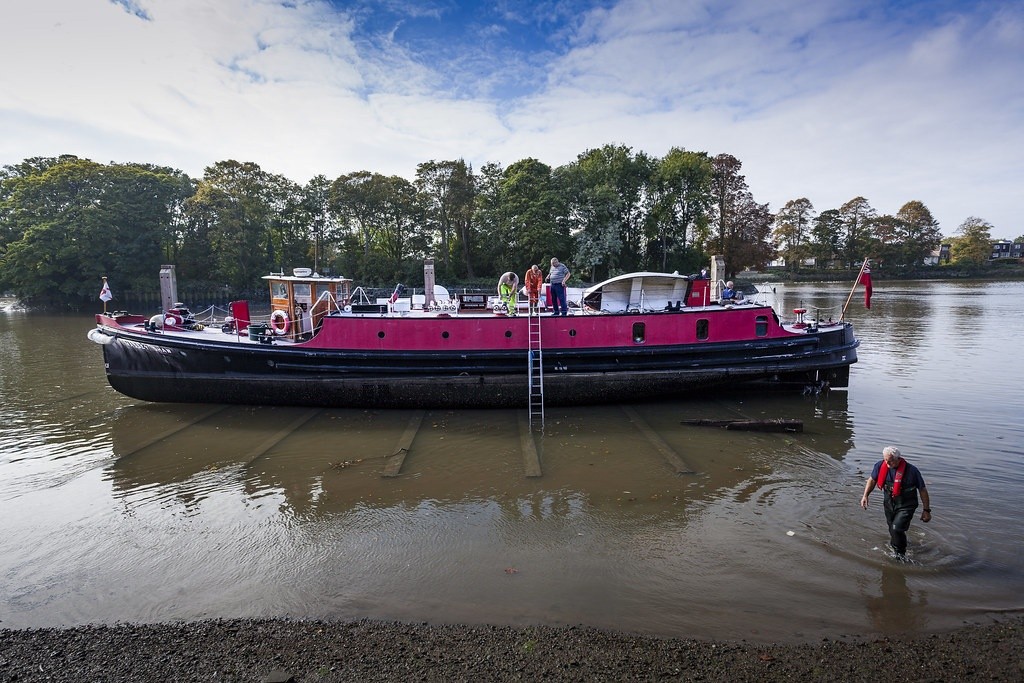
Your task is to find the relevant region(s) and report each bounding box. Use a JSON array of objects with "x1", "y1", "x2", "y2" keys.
[
  {"x1": 551, "y1": 313, "x2": 559, "y2": 316},
  {"x1": 562, "y1": 314, "x2": 566, "y2": 316}
]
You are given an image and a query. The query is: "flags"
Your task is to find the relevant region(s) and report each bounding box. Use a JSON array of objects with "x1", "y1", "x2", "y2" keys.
[
  {"x1": 100, "y1": 282, "x2": 112, "y2": 303},
  {"x1": 858, "y1": 260, "x2": 873, "y2": 310}
]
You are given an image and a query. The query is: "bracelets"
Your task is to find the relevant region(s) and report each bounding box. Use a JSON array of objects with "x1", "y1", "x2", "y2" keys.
[{"x1": 922, "y1": 508, "x2": 931, "y2": 512}]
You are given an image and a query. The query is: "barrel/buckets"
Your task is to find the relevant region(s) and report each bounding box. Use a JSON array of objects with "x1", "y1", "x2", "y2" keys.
[{"x1": 247, "y1": 325, "x2": 266, "y2": 340}]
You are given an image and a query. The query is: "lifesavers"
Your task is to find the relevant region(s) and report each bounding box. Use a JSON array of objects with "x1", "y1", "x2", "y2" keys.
[{"x1": 268, "y1": 310, "x2": 289, "y2": 336}]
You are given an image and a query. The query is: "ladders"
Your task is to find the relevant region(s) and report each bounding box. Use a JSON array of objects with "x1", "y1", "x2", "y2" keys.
[{"x1": 528, "y1": 290, "x2": 545, "y2": 437}]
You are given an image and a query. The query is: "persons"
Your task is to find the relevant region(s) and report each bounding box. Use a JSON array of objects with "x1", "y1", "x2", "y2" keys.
[
  {"x1": 524, "y1": 265, "x2": 542, "y2": 316},
  {"x1": 497, "y1": 271, "x2": 519, "y2": 317},
  {"x1": 721, "y1": 280, "x2": 750, "y2": 305},
  {"x1": 545, "y1": 258, "x2": 570, "y2": 316},
  {"x1": 701, "y1": 268, "x2": 707, "y2": 279},
  {"x1": 860, "y1": 446, "x2": 932, "y2": 562}
]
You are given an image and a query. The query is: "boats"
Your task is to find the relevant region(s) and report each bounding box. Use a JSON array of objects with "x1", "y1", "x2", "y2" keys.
[{"x1": 86, "y1": 218, "x2": 861, "y2": 410}]
